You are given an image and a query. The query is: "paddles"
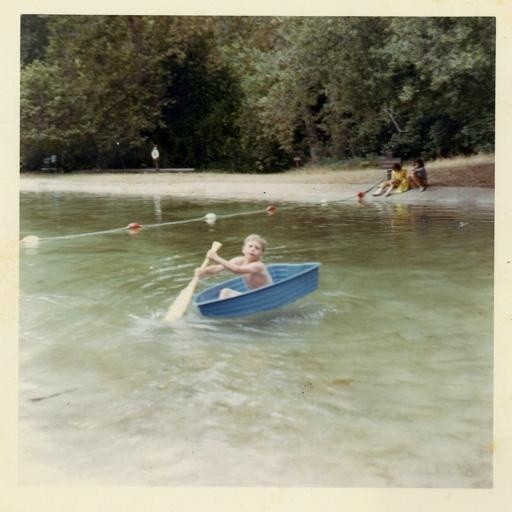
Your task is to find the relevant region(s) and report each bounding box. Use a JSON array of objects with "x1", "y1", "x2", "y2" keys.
[{"x1": 168, "y1": 241, "x2": 223, "y2": 321}]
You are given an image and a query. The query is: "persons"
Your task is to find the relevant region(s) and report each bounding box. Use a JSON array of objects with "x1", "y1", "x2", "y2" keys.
[
  {"x1": 194, "y1": 233, "x2": 274, "y2": 298},
  {"x1": 372, "y1": 162, "x2": 409, "y2": 198},
  {"x1": 406, "y1": 157, "x2": 428, "y2": 192}
]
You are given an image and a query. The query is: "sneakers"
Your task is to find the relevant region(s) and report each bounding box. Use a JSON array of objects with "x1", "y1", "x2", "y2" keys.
[{"x1": 372, "y1": 186, "x2": 392, "y2": 196}]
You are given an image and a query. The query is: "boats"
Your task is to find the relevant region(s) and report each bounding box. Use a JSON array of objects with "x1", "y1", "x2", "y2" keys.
[{"x1": 195, "y1": 259, "x2": 319, "y2": 321}]
